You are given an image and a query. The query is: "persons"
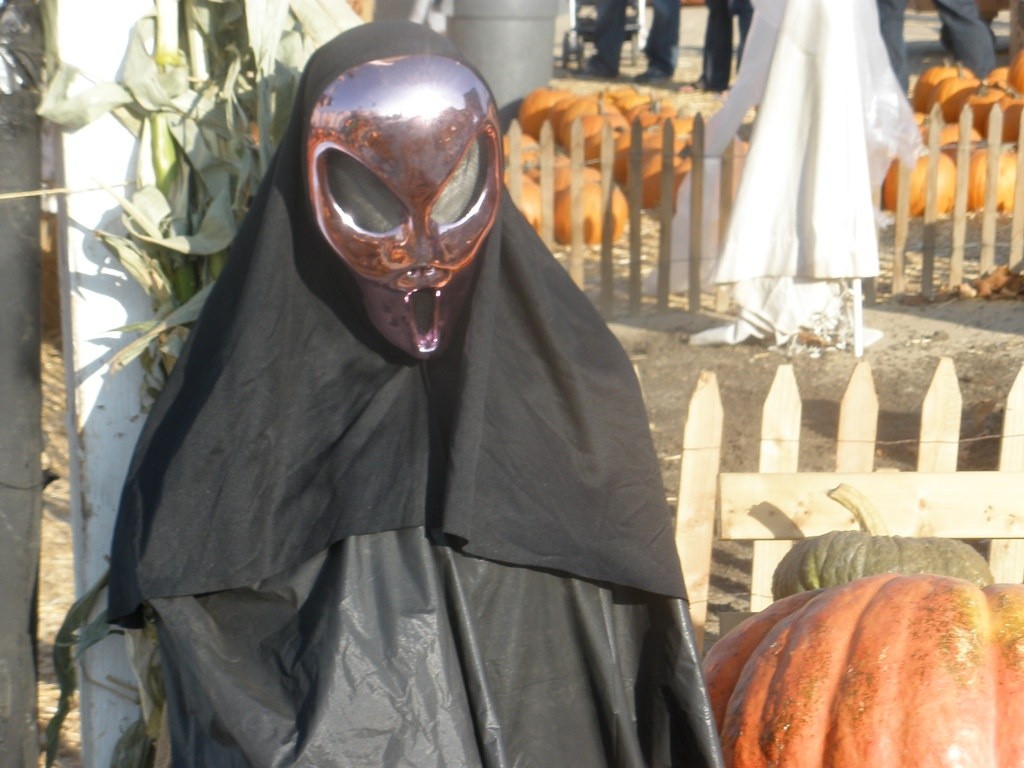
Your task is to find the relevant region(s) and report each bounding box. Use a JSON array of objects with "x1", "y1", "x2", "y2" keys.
[
  {"x1": 874, "y1": 1, "x2": 998, "y2": 100},
  {"x1": 584, "y1": 0, "x2": 683, "y2": 86},
  {"x1": 692, "y1": 1, "x2": 756, "y2": 94}
]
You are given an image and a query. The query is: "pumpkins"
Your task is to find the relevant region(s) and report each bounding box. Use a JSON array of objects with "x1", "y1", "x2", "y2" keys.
[
  {"x1": 699, "y1": 484, "x2": 1024, "y2": 768},
  {"x1": 499, "y1": 84, "x2": 745, "y2": 245},
  {"x1": 878, "y1": 47, "x2": 1024, "y2": 220}
]
